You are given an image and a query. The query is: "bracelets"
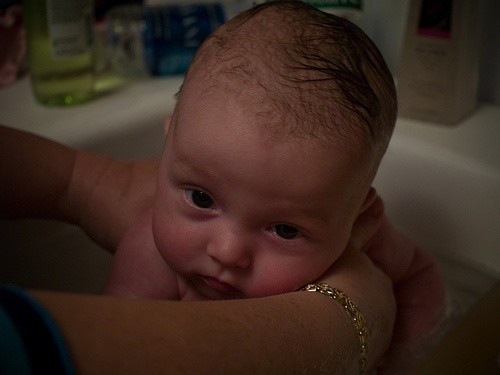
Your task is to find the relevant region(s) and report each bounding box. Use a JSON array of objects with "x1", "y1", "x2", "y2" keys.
[{"x1": 300, "y1": 283, "x2": 369, "y2": 375}]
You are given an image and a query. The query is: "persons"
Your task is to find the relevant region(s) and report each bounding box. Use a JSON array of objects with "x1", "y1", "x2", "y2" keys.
[
  {"x1": 1, "y1": 2, "x2": 446, "y2": 375},
  {"x1": 0, "y1": 125, "x2": 398, "y2": 375}
]
